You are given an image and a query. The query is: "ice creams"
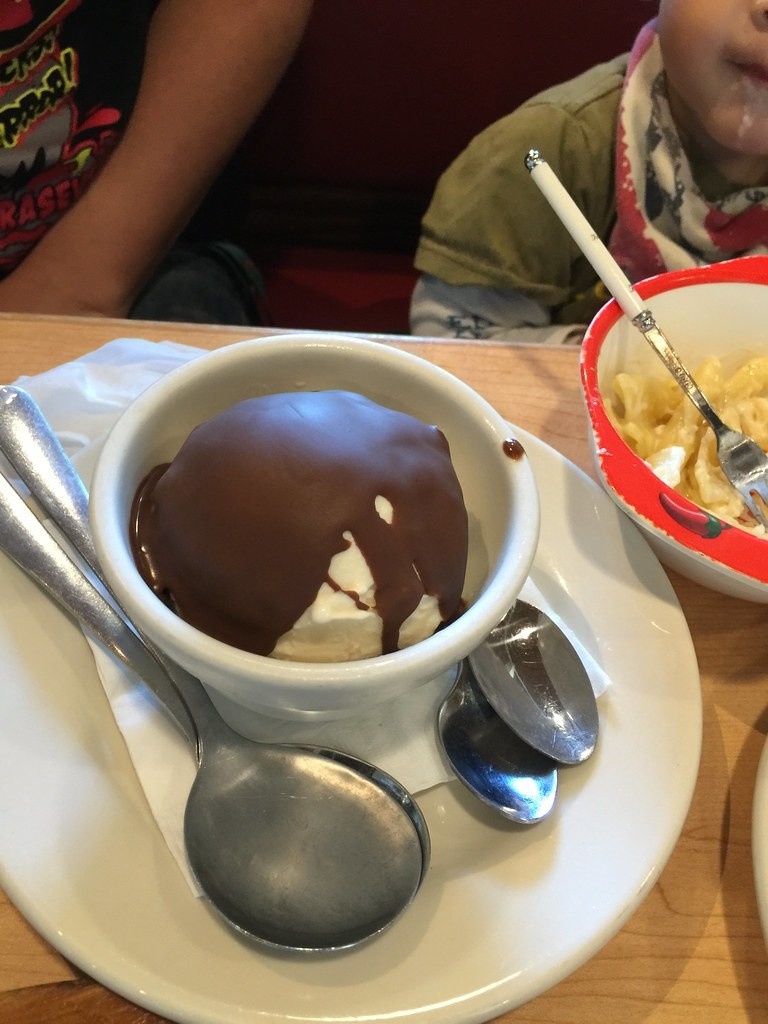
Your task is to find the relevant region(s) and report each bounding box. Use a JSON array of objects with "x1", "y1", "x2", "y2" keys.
[{"x1": 130, "y1": 390, "x2": 468, "y2": 664}]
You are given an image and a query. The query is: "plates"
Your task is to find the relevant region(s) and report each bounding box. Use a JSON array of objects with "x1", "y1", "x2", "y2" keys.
[{"x1": 0, "y1": 419, "x2": 703, "y2": 1022}]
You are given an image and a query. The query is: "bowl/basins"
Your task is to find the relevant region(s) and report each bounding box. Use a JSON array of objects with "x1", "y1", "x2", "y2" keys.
[{"x1": 578, "y1": 252, "x2": 768, "y2": 607}]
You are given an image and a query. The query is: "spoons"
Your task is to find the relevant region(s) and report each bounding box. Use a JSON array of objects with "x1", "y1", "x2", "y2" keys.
[
  {"x1": 468, "y1": 598, "x2": 598, "y2": 767},
  {"x1": 437, "y1": 660, "x2": 560, "y2": 824},
  {"x1": 1, "y1": 472, "x2": 431, "y2": 903},
  {"x1": 0, "y1": 385, "x2": 422, "y2": 952}
]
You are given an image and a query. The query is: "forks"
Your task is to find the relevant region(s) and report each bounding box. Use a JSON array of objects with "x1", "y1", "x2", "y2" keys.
[{"x1": 525, "y1": 149, "x2": 768, "y2": 530}]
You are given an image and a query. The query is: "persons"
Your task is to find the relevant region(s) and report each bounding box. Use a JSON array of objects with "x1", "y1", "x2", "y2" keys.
[
  {"x1": 410, "y1": 2, "x2": 768, "y2": 350},
  {"x1": 0, "y1": 0, "x2": 323, "y2": 319}
]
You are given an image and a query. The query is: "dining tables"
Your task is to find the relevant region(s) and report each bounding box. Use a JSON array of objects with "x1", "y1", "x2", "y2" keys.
[{"x1": 0, "y1": 311, "x2": 768, "y2": 1023}]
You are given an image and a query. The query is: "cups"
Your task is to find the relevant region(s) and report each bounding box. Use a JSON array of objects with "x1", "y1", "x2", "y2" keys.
[{"x1": 91, "y1": 335, "x2": 542, "y2": 723}]
